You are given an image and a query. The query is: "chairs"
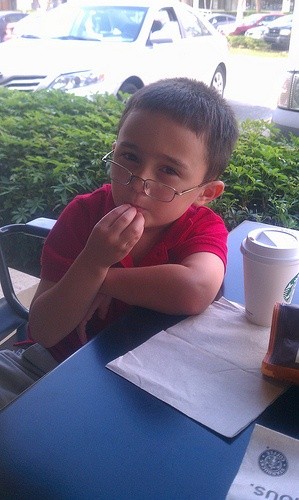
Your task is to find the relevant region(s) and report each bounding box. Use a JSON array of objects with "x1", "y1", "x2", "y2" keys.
[{"x1": 0, "y1": 224, "x2": 93, "y2": 372}]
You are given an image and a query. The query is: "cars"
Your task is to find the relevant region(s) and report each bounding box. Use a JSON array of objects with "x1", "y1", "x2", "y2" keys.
[
  {"x1": 0, "y1": 9, "x2": 31, "y2": 44},
  {"x1": 201, "y1": 12, "x2": 294, "y2": 52},
  {"x1": 0, "y1": 0, "x2": 232, "y2": 103}
]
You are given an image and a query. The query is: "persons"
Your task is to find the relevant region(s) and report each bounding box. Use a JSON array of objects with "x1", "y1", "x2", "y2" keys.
[{"x1": 25, "y1": 77, "x2": 240, "y2": 349}]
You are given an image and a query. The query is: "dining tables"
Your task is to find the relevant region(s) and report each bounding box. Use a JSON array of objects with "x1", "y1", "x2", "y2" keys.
[{"x1": 0, "y1": 221, "x2": 299, "y2": 500}]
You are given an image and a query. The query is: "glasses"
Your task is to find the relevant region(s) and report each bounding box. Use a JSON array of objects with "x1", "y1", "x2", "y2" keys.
[{"x1": 102, "y1": 150, "x2": 221, "y2": 203}]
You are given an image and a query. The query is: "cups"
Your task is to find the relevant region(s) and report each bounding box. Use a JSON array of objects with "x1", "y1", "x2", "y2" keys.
[{"x1": 240, "y1": 226, "x2": 299, "y2": 325}]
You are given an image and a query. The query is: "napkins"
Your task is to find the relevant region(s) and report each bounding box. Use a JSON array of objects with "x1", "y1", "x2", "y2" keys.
[{"x1": 105, "y1": 297, "x2": 284, "y2": 437}]
